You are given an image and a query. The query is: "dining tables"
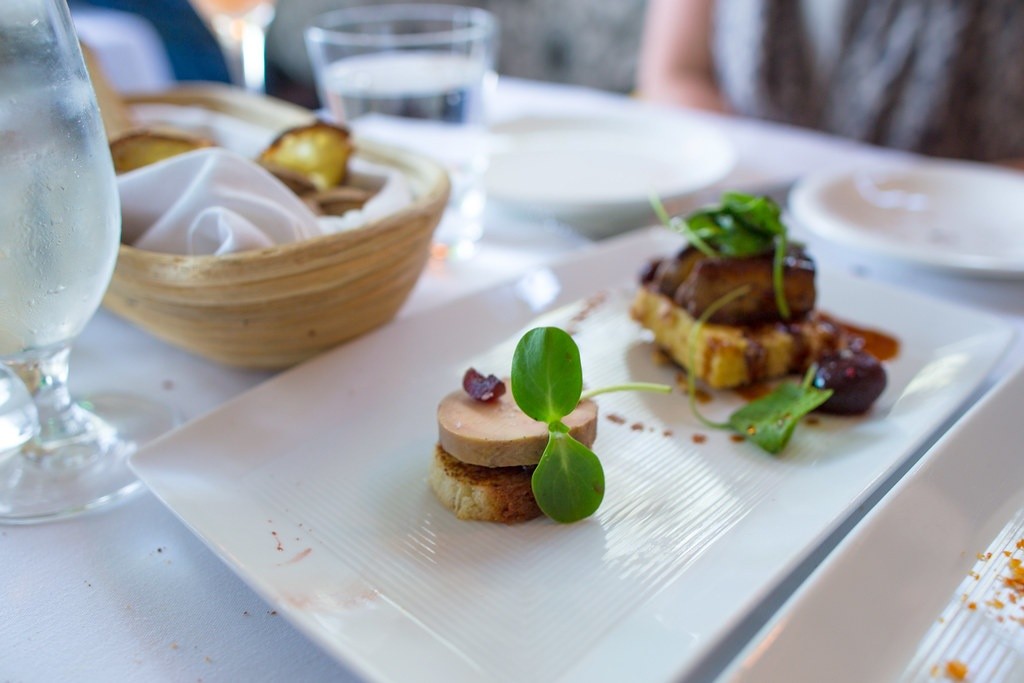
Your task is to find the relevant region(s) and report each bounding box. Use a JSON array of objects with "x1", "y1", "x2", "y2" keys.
[{"x1": 0, "y1": 73, "x2": 1023, "y2": 681}]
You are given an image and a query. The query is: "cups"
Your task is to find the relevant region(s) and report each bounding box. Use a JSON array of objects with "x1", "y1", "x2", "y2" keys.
[{"x1": 306, "y1": 4, "x2": 502, "y2": 256}]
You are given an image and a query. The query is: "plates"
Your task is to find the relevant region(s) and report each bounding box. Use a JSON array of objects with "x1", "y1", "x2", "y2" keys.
[
  {"x1": 475, "y1": 107, "x2": 734, "y2": 235},
  {"x1": 787, "y1": 153, "x2": 1023, "y2": 277},
  {"x1": 712, "y1": 361, "x2": 1023, "y2": 682},
  {"x1": 122, "y1": 223, "x2": 1012, "y2": 682}
]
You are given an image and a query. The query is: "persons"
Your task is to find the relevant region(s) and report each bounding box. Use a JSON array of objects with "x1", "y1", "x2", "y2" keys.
[{"x1": 636, "y1": 0, "x2": 1024, "y2": 170}]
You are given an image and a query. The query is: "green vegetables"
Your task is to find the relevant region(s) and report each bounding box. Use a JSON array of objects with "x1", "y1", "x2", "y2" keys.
[
  {"x1": 652, "y1": 195, "x2": 791, "y2": 319},
  {"x1": 688, "y1": 284, "x2": 833, "y2": 453},
  {"x1": 511, "y1": 328, "x2": 673, "y2": 525}
]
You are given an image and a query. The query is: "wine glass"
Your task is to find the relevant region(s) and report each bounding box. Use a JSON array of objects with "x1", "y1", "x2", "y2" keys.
[{"x1": 1, "y1": 0, "x2": 182, "y2": 523}]
